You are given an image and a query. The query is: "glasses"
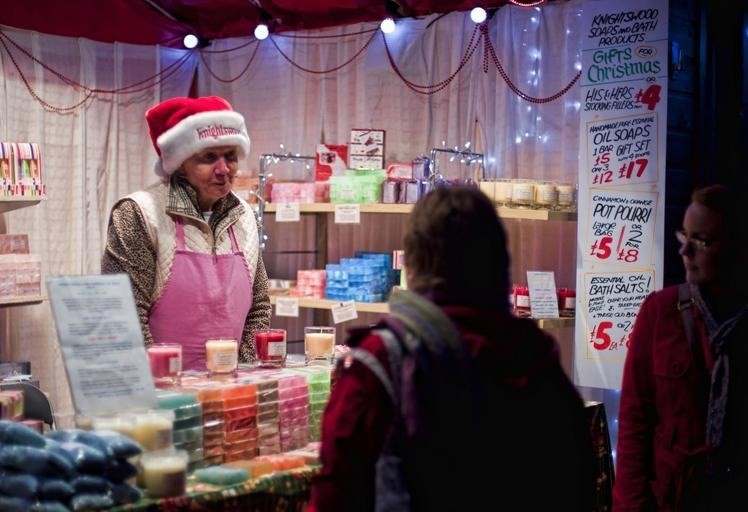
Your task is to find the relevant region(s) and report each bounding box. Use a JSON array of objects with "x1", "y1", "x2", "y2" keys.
[{"x1": 674, "y1": 231, "x2": 712, "y2": 254}]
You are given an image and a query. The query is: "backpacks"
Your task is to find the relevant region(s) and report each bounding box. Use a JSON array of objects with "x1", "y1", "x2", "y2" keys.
[{"x1": 372, "y1": 314, "x2": 599, "y2": 512}]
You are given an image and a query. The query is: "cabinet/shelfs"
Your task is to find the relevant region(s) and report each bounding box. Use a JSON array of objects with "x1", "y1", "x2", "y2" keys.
[
  {"x1": 247, "y1": 200, "x2": 578, "y2": 332},
  {"x1": 0, "y1": 194, "x2": 48, "y2": 309}
]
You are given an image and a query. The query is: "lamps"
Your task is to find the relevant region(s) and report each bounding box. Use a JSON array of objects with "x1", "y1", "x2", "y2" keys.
[{"x1": 146, "y1": 0, "x2": 511, "y2": 51}]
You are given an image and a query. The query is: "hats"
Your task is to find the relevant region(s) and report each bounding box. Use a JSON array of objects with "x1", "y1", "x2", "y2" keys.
[{"x1": 145, "y1": 96, "x2": 250, "y2": 177}]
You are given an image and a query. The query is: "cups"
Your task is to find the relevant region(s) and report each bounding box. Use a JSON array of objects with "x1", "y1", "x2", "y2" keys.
[
  {"x1": 141, "y1": 449, "x2": 188, "y2": 496},
  {"x1": 146, "y1": 326, "x2": 336, "y2": 390}
]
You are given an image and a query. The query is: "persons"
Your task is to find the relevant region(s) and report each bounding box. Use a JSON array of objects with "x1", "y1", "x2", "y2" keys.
[
  {"x1": 609, "y1": 183, "x2": 747, "y2": 511},
  {"x1": 307, "y1": 185, "x2": 600, "y2": 512},
  {"x1": 99, "y1": 96, "x2": 273, "y2": 373}
]
